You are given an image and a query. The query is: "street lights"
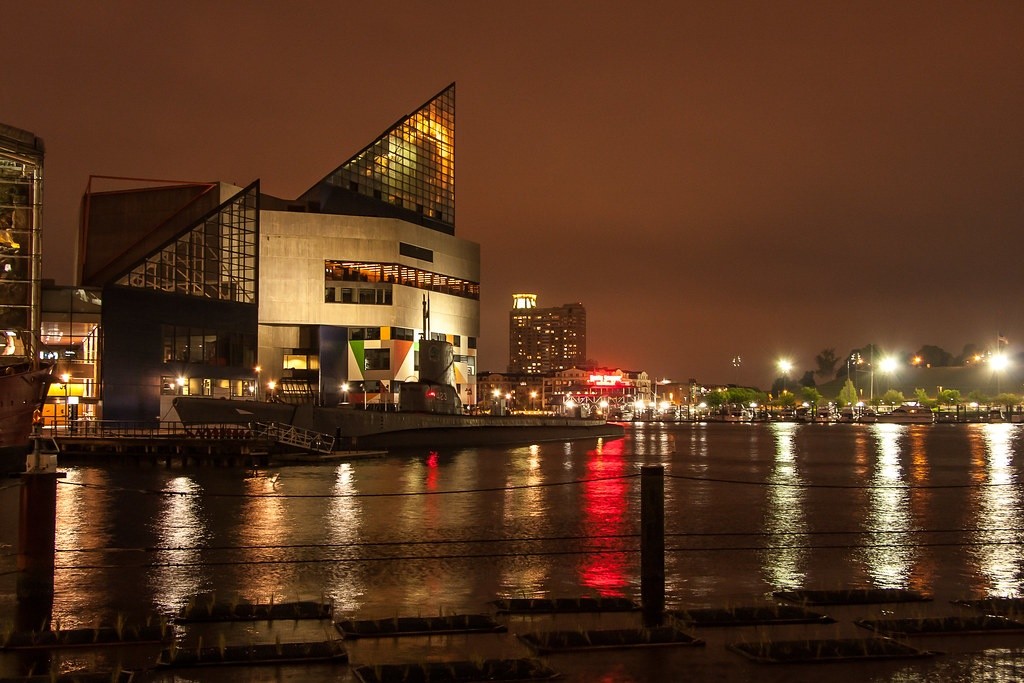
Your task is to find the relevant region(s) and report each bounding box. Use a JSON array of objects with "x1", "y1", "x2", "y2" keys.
[
  {"x1": 255, "y1": 366, "x2": 261, "y2": 401},
  {"x1": 777, "y1": 358, "x2": 793, "y2": 390},
  {"x1": 985, "y1": 349, "x2": 1017, "y2": 393},
  {"x1": 60, "y1": 373, "x2": 71, "y2": 434},
  {"x1": 342, "y1": 384, "x2": 350, "y2": 402},
  {"x1": 877, "y1": 353, "x2": 901, "y2": 391}
]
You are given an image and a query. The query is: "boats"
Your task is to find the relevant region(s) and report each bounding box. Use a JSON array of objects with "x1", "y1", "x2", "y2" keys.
[{"x1": 0, "y1": 356, "x2": 59, "y2": 474}]
